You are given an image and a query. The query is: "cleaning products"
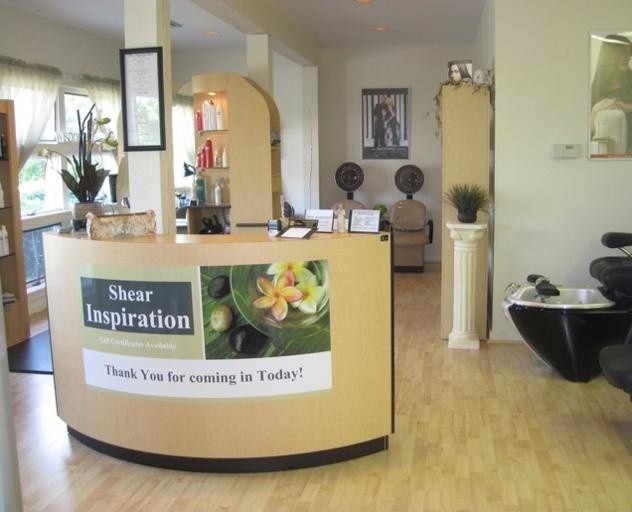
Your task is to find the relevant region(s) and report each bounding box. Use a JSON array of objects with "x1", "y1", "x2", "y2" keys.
[
  {"x1": 213, "y1": 177, "x2": 225, "y2": 206},
  {"x1": 196, "y1": 99, "x2": 223, "y2": 130},
  {"x1": 195, "y1": 137, "x2": 228, "y2": 168}
]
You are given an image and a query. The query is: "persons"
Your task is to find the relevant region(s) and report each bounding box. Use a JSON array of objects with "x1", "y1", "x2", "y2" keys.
[
  {"x1": 449, "y1": 64, "x2": 469, "y2": 79},
  {"x1": 591, "y1": 34, "x2": 632, "y2": 138},
  {"x1": 372, "y1": 96, "x2": 401, "y2": 147}
]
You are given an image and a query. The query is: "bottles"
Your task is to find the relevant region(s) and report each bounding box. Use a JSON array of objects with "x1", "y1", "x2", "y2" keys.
[
  {"x1": 337, "y1": 205, "x2": 345, "y2": 234},
  {"x1": 0, "y1": 132, "x2": 10, "y2": 257}
]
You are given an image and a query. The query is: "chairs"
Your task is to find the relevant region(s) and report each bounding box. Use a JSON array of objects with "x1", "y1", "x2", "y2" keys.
[{"x1": 333, "y1": 200, "x2": 433, "y2": 273}]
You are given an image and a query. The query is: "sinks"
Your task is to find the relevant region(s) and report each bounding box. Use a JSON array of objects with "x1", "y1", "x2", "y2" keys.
[{"x1": 503, "y1": 282, "x2": 631, "y2": 382}]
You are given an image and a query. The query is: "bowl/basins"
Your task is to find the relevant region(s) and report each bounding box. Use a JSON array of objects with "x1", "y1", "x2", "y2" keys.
[{"x1": 230, "y1": 260, "x2": 330, "y2": 341}]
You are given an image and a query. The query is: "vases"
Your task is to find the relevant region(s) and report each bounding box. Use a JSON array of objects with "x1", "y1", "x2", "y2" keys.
[{"x1": 74, "y1": 201, "x2": 102, "y2": 223}]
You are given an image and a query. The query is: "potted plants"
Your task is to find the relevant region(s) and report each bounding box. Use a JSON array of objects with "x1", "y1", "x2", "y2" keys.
[{"x1": 441, "y1": 185, "x2": 492, "y2": 224}]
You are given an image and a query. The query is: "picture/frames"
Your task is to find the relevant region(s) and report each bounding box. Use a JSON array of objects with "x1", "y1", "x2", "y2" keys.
[{"x1": 120, "y1": 45, "x2": 164, "y2": 152}]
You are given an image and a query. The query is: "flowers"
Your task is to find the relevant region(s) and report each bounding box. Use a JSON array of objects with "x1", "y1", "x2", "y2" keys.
[{"x1": 38, "y1": 104, "x2": 119, "y2": 203}]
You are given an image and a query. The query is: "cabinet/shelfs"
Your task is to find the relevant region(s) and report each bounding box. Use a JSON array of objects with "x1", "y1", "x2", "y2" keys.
[
  {"x1": 440, "y1": 88, "x2": 490, "y2": 340},
  {"x1": 197, "y1": 129, "x2": 230, "y2": 207},
  {"x1": 1, "y1": 99, "x2": 32, "y2": 348}
]
